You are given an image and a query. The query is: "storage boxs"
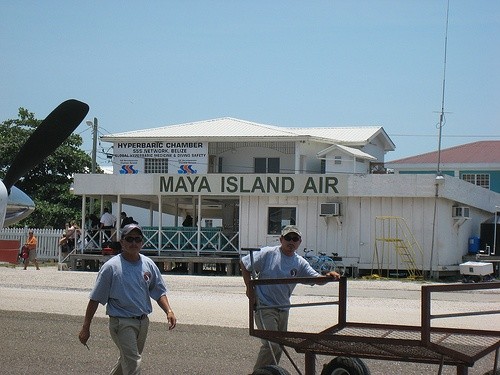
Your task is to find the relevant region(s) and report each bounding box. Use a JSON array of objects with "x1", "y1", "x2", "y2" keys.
[{"x1": 460, "y1": 261, "x2": 494, "y2": 276}]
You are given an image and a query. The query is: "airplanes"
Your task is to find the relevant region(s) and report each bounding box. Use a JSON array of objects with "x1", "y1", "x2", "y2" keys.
[{"x1": 0, "y1": 99, "x2": 90, "y2": 238}]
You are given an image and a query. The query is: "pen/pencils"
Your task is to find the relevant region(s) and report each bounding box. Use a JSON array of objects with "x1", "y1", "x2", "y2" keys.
[{"x1": 85, "y1": 343, "x2": 90, "y2": 350}]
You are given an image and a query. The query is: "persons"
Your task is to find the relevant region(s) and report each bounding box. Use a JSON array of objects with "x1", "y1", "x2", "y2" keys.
[
  {"x1": 21, "y1": 208, "x2": 194, "y2": 270},
  {"x1": 79, "y1": 223, "x2": 176, "y2": 375},
  {"x1": 240, "y1": 225, "x2": 341, "y2": 375}
]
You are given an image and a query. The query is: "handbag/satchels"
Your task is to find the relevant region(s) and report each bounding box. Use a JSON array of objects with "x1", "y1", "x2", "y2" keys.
[{"x1": 22, "y1": 245, "x2": 30, "y2": 258}]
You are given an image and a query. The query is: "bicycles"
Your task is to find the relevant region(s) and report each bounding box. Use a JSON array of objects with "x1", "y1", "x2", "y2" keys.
[{"x1": 302, "y1": 249, "x2": 346, "y2": 277}]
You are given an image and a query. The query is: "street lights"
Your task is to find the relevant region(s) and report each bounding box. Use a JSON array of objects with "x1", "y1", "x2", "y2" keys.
[{"x1": 86, "y1": 117, "x2": 98, "y2": 224}]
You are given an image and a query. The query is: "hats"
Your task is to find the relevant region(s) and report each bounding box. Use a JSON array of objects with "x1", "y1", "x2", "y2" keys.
[
  {"x1": 121, "y1": 223, "x2": 144, "y2": 237},
  {"x1": 281, "y1": 225, "x2": 302, "y2": 237}
]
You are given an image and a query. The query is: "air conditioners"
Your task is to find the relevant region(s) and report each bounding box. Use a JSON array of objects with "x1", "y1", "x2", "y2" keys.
[
  {"x1": 452, "y1": 207, "x2": 470, "y2": 219},
  {"x1": 320, "y1": 203, "x2": 340, "y2": 215}
]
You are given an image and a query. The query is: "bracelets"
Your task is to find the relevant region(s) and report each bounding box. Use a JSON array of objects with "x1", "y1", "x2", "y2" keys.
[{"x1": 166, "y1": 309, "x2": 173, "y2": 314}]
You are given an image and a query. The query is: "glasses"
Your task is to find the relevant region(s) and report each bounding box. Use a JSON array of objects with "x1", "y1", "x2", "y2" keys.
[
  {"x1": 122, "y1": 236, "x2": 142, "y2": 242},
  {"x1": 283, "y1": 236, "x2": 299, "y2": 242}
]
